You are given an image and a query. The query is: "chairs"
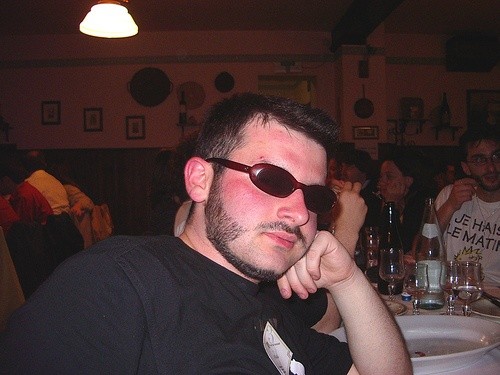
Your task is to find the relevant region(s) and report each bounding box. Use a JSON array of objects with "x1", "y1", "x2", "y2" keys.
[{"x1": 7, "y1": 203, "x2": 113, "y2": 293}]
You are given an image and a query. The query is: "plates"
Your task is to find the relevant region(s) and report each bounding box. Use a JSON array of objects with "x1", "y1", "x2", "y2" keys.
[
  {"x1": 328, "y1": 315, "x2": 500, "y2": 375},
  {"x1": 384, "y1": 298, "x2": 408, "y2": 316},
  {"x1": 469, "y1": 298, "x2": 500, "y2": 319}
]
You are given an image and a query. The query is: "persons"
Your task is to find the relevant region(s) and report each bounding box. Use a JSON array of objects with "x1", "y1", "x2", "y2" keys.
[
  {"x1": 173, "y1": 128, "x2": 368, "y2": 333},
  {"x1": 320, "y1": 147, "x2": 457, "y2": 268},
  {"x1": 0, "y1": 149, "x2": 95, "y2": 241},
  {"x1": 434, "y1": 122, "x2": 500, "y2": 296},
  {"x1": 0, "y1": 92, "x2": 414, "y2": 375}
]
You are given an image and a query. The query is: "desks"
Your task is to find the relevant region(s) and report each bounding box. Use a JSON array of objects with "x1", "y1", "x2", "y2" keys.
[{"x1": 339, "y1": 252, "x2": 500, "y2": 375}]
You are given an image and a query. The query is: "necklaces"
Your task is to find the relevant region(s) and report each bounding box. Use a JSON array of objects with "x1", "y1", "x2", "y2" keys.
[{"x1": 475, "y1": 195, "x2": 500, "y2": 229}]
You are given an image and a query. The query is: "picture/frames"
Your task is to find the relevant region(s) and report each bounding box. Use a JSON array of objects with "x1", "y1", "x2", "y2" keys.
[
  {"x1": 353, "y1": 126, "x2": 379, "y2": 139},
  {"x1": 41, "y1": 101, "x2": 60, "y2": 125},
  {"x1": 125, "y1": 115, "x2": 146, "y2": 139},
  {"x1": 83, "y1": 108, "x2": 103, "y2": 132},
  {"x1": 386, "y1": 119, "x2": 397, "y2": 143},
  {"x1": 467, "y1": 89, "x2": 500, "y2": 130}
]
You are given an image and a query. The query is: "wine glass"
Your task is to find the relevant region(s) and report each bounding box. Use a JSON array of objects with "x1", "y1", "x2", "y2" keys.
[
  {"x1": 378, "y1": 248, "x2": 429, "y2": 315},
  {"x1": 439, "y1": 260, "x2": 483, "y2": 317}
]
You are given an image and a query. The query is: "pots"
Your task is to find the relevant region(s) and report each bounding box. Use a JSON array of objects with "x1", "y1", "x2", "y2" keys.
[
  {"x1": 127, "y1": 67, "x2": 174, "y2": 107},
  {"x1": 354, "y1": 83, "x2": 375, "y2": 119}
]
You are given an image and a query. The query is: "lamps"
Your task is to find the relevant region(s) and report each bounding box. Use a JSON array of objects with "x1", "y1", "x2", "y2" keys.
[{"x1": 79, "y1": 0, "x2": 139, "y2": 39}]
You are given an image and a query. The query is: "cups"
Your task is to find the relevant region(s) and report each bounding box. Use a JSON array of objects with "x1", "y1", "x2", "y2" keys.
[{"x1": 364, "y1": 225, "x2": 380, "y2": 267}]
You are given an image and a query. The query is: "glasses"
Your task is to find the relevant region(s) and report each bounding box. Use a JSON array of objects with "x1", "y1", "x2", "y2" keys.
[
  {"x1": 204, "y1": 158, "x2": 337, "y2": 216},
  {"x1": 465, "y1": 153, "x2": 500, "y2": 165}
]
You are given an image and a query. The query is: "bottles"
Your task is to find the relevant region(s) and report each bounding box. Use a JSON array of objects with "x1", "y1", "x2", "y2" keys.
[
  {"x1": 376, "y1": 201, "x2": 404, "y2": 295},
  {"x1": 415, "y1": 198, "x2": 446, "y2": 310},
  {"x1": 441, "y1": 92, "x2": 450, "y2": 117},
  {"x1": 178, "y1": 91, "x2": 187, "y2": 124}
]
